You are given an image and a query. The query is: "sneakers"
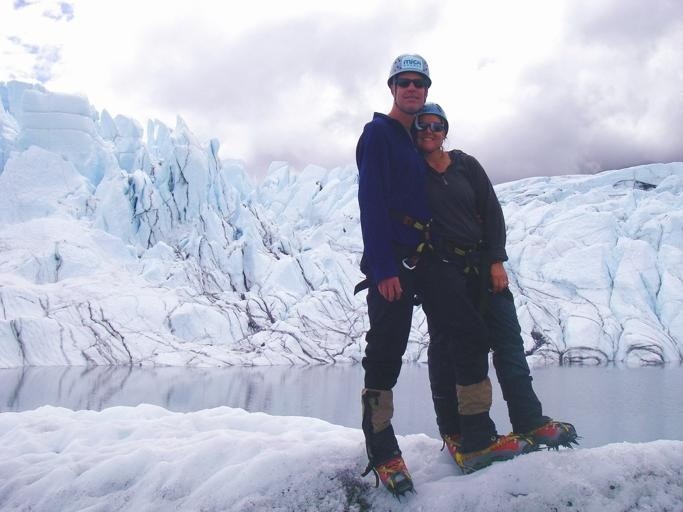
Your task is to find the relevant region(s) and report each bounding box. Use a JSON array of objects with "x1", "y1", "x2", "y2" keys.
[
  {"x1": 435, "y1": 419, "x2": 578, "y2": 476},
  {"x1": 363, "y1": 450, "x2": 419, "y2": 503}
]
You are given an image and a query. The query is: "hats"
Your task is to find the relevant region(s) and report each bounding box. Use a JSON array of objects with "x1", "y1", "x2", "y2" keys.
[
  {"x1": 387, "y1": 53, "x2": 432, "y2": 88},
  {"x1": 413, "y1": 102, "x2": 450, "y2": 131}
]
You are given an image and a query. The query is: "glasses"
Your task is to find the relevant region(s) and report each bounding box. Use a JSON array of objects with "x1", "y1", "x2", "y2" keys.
[
  {"x1": 413, "y1": 121, "x2": 445, "y2": 134},
  {"x1": 393, "y1": 77, "x2": 428, "y2": 89}
]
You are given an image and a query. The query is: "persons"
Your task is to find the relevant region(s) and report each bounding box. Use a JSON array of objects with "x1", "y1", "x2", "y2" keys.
[
  {"x1": 407, "y1": 102, "x2": 579, "y2": 472},
  {"x1": 354, "y1": 52, "x2": 502, "y2": 496}
]
